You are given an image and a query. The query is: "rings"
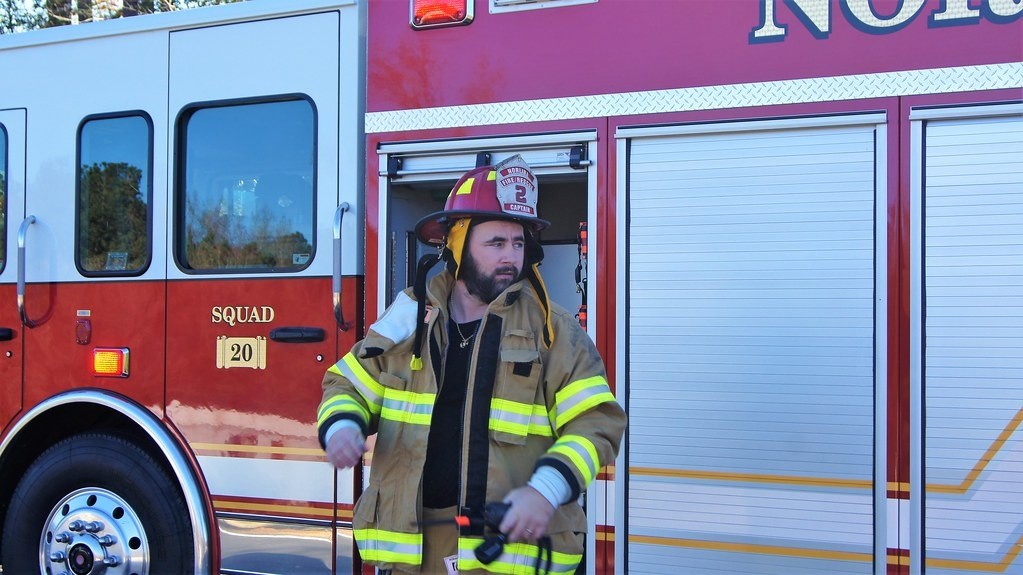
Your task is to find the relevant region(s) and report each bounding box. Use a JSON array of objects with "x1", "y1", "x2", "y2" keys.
[{"x1": 525, "y1": 528, "x2": 533, "y2": 534}]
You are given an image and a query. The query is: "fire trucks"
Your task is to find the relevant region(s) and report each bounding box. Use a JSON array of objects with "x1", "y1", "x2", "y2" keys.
[{"x1": 0, "y1": 0, "x2": 1023, "y2": 575}]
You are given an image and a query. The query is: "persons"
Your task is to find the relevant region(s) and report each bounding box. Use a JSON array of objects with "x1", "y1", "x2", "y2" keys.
[{"x1": 318, "y1": 162, "x2": 626, "y2": 575}]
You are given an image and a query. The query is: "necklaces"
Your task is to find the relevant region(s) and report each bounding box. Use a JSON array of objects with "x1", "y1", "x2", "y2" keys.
[{"x1": 450, "y1": 293, "x2": 474, "y2": 348}]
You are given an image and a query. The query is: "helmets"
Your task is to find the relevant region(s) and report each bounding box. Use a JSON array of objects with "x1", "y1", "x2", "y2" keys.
[{"x1": 413, "y1": 153, "x2": 551, "y2": 249}]
